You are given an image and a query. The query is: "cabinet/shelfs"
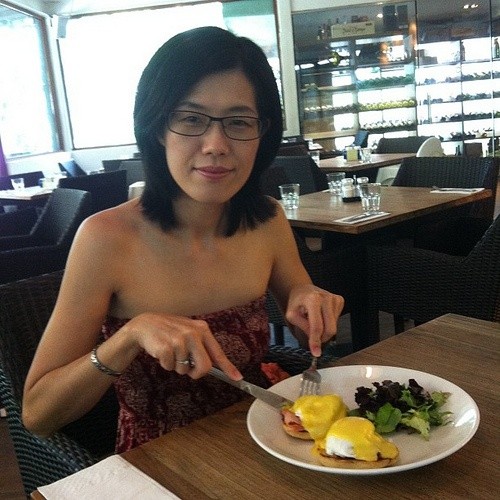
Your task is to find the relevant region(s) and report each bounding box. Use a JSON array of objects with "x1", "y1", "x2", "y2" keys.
[{"x1": 292, "y1": 16, "x2": 500, "y2": 156}]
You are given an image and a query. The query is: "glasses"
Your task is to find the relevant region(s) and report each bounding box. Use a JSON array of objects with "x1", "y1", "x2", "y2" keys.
[{"x1": 166, "y1": 109, "x2": 263, "y2": 141}]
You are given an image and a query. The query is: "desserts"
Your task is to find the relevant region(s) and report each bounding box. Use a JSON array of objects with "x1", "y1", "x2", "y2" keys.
[
  {"x1": 280, "y1": 392, "x2": 349, "y2": 441},
  {"x1": 311, "y1": 416, "x2": 401, "y2": 468}
]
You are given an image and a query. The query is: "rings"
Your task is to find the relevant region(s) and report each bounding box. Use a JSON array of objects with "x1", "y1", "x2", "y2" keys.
[{"x1": 174, "y1": 360, "x2": 192, "y2": 365}]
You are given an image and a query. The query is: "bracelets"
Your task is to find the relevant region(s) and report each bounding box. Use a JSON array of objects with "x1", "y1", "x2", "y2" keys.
[{"x1": 91, "y1": 343, "x2": 123, "y2": 378}]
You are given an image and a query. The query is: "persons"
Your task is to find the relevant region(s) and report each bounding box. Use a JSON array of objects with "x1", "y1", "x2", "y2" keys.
[{"x1": 22, "y1": 27, "x2": 344, "y2": 454}]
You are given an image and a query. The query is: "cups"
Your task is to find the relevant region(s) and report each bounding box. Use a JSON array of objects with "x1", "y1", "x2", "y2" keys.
[
  {"x1": 279, "y1": 183, "x2": 300, "y2": 210},
  {"x1": 326, "y1": 172, "x2": 345, "y2": 194},
  {"x1": 53, "y1": 171, "x2": 67, "y2": 189},
  {"x1": 347, "y1": 147, "x2": 358, "y2": 162},
  {"x1": 11, "y1": 178, "x2": 24, "y2": 192},
  {"x1": 360, "y1": 148, "x2": 372, "y2": 163},
  {"x1": 310, "y1": 151, "x2": 319, "y2": 165},
  {"x1": 358, "y1": 182, "x2": 382, "y2": 212}
]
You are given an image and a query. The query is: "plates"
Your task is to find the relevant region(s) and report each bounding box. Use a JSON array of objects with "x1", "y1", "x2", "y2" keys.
[{"x1": 246, "y1": 365, "x2": 482, "y2": 477}]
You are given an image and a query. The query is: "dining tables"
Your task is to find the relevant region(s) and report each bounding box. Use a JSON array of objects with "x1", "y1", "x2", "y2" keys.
[
  {"x1": 0, "y1": 186, "x2": 54, "y2": 201},
  {"x1": 30, "y1": 312, "x2": 500, "y2": 500},
  {"x1": 278, "y1": 186, "x2": 492, "y2": 234},
  {"x1": 314, "y1": 153, "x2": 415, "y2": 172}
]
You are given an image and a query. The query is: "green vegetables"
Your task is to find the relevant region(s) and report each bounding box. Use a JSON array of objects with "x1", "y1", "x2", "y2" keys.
[{"x1": 346, "y1": 386, "x2": 454, "y2": 441}]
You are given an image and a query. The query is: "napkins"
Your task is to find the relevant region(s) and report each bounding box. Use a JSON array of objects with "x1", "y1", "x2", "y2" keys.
[
  {"x1": 37, "y1": 453, "x2": 182, "y2": 499},
  {"x1": 429, "y1": 187, "x2": 486, "y2": 195}
]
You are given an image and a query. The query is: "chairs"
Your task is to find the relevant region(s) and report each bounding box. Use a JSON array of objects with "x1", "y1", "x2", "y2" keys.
[{"x1": 0, "y1": 142, "x2": 500, "y2": 500}]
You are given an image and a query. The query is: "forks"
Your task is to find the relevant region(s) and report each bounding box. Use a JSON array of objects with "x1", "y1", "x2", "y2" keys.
[{"x1": 299, "y1": 356, "x2": 321, "y2": 398}]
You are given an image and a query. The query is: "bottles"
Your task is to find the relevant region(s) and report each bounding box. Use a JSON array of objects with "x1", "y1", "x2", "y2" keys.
[
  {"x1": 455, "y1": 145, "x2": 461, "y2": 156},
  {"x1": 317, "y1": 15, "x2": 348, "y2": 40},
  {"x1": 494, "y1": 39, "x2": 499, "y2": 58},
  {"x1": 342, "y1": 178, "x2": 355, "y2": 197},
  {"x1": 357, "y1": 177, "x2": 369, "y2": 197},
  {"x1": 461, "y1": 43, "x2": 465, "y2": 61}
]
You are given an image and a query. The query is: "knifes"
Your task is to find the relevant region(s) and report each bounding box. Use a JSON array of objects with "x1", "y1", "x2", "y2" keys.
[{"x1": 189, "y1": 360, "x2": 295, "y2": 410}]
